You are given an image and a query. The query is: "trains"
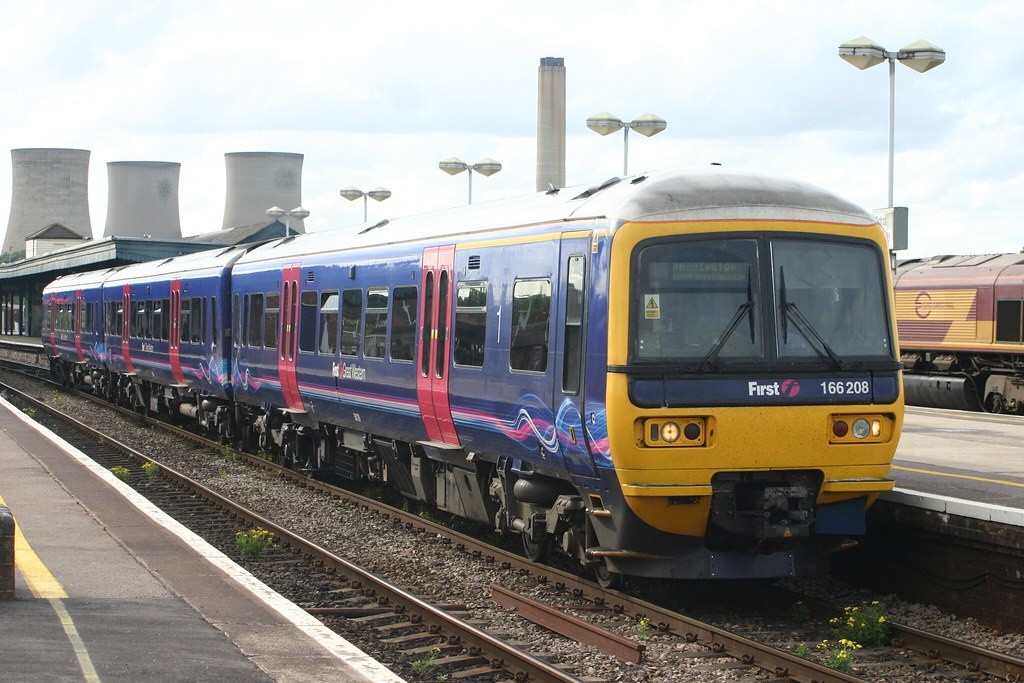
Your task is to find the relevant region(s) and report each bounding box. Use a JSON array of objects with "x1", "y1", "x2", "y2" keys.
[
  {"x1": 891, "y1": 247, "x2": 1024, "y2": 416},
  {"x1": 42, "y1": 163, "x2": 904, "y2": 588}
]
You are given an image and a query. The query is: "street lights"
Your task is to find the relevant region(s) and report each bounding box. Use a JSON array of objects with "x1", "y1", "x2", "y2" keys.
[
  {"x1": 266, "y1": 206, "x2": 310, "y2": 237},
  {"x1": 340, "y1": 185, "x2": 391, "y2": 223},
  {"x1": 439, "y1": 156, "x2": 502, "y2": 205},
  {"x1": 586, "y1": 111, "x2": 667, "y2": 176},
  {"x1": 839, "y1": 36, "x2": 946, "y2": 275}
]
